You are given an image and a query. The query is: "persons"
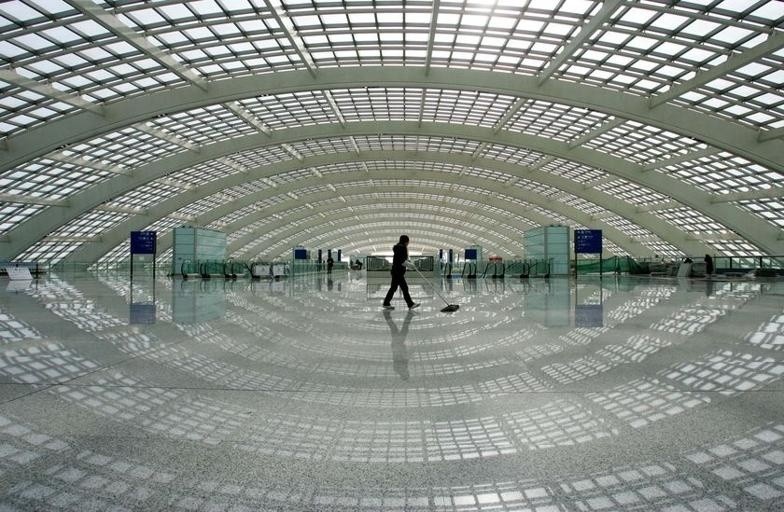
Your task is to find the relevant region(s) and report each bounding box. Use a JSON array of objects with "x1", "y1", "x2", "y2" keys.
[
  {"x1": 382, "y1": 235, "x2": 421, "y2": 310},
  {"x1": 327, "y1": 254, "x2": 335, "y2": 274},
  {"x1": 350, "y1": 259, "x2": 362, "y2": 270},
  {"x1": 704, "y1": 254, "x2": 714, "y2": 279},
  {"x1": 328, "y1": 279, "x2": 333, "y2": 291},
  {"x1": 684, "y1": 257, "x2": 692, "y2": 264}
]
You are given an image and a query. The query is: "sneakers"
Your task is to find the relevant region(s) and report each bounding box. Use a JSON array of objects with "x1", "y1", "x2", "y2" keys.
[
  {"x1": 383, "y1": 305, "x2": 394, "y2": 309},
  {"x1": 409, "y1": 304, "x2": 420, "y2": 310}
]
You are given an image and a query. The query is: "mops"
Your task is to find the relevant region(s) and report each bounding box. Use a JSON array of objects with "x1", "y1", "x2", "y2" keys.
[{"x1": 410, "y1": 261, "x2": 460, "y2": 312}]
